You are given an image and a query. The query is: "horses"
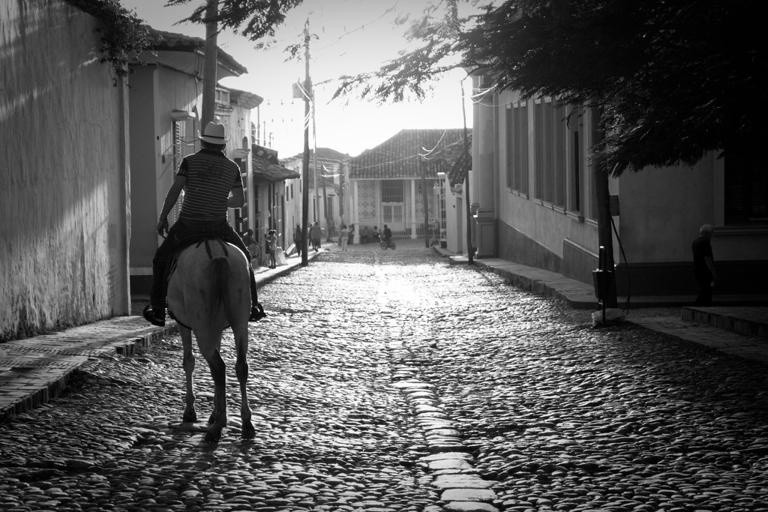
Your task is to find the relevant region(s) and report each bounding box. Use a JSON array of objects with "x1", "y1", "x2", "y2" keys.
[{"x1": 165, "y1": 234, "x2": 259, "y2": 446}]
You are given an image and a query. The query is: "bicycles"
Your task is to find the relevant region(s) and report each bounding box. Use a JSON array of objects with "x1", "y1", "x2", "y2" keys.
[{"x1": 380, "y1": 239, "x2": 395, "y2": 250}]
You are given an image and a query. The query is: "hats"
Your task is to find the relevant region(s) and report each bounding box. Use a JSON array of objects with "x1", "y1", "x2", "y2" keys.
[{"x1": 197, "y1": 121, "x2": 230, "y2": 144}]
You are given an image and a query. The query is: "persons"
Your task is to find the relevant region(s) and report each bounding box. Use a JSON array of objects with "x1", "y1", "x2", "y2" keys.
[
  {"x1": 293, "y1": 224, "x2": 303, "y2": 256},
  {"x1": 308, "y1": 224, "x2": 314, "y2": 246},
  {"x1": 336, "y1": 225, "x2": 351, "y2": 251},
  {"x1": 142, "y1": 119, "x2": 266, "y2": 327},
  {"x1": 243, "y1": 228, "x2": 259, "y2": 267},
  {"x1": 265, "y1": 233, "x2": 272, "y2": 257},
  {"x1": 266, "y1": 229, "x2": 279, "y2": 269},
  {"x1": 311, "y1": 221, "x2": 320, "y2": 252},
  {"x1": 382, "y1": 224, "x2": 392, "y2": 249}
]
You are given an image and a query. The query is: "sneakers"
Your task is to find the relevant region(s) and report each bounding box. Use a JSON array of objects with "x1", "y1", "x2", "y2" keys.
[
  {"x1": 251, "y1": 305, "x2": 268, "y2": 318},
  {"x1": 268, "y1": 264, "x2": 277, "y2": 269},
  {"x1": 142, "y1": 305, "x2": 166, "y2": 326}
]
